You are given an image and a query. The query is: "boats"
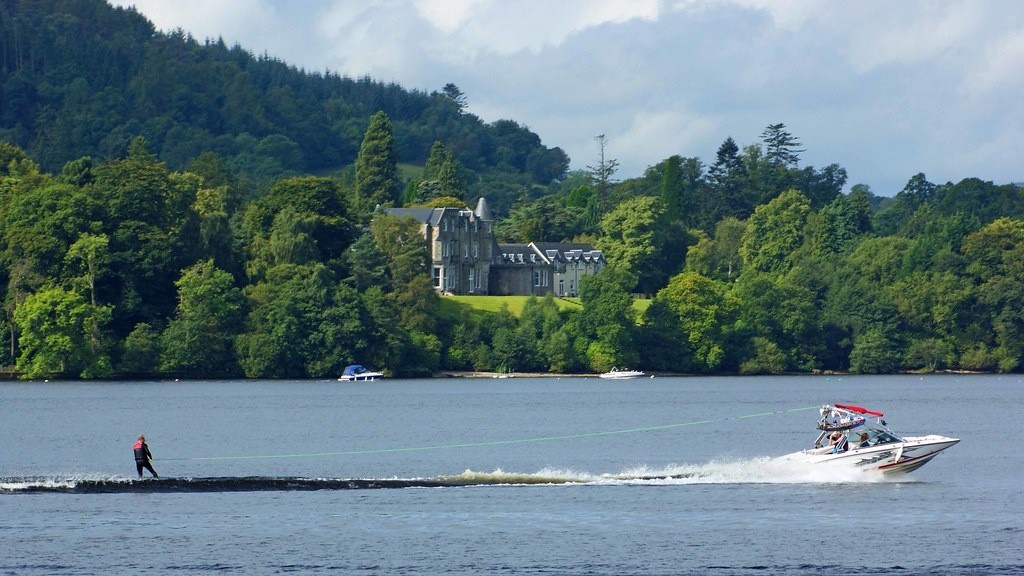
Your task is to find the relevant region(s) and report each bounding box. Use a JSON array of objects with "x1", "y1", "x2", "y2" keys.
[
  {"x1": 337, "y1": 365, "x2": 383, "y2": 381},
  {"x1": 599, "y1": 366, "x2": 645, "y2": 380},
  {"x1": 777, "y1": 404, "x2": 961, "y2": 481}
]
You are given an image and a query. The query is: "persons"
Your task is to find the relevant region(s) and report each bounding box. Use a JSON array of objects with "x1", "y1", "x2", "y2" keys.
[
  {"x1": 133, "y1": 435, "x2": 159, "y2": 478},
  {"x1": 826, "y1": 430, "x2": 869, "y2": 450}
]
